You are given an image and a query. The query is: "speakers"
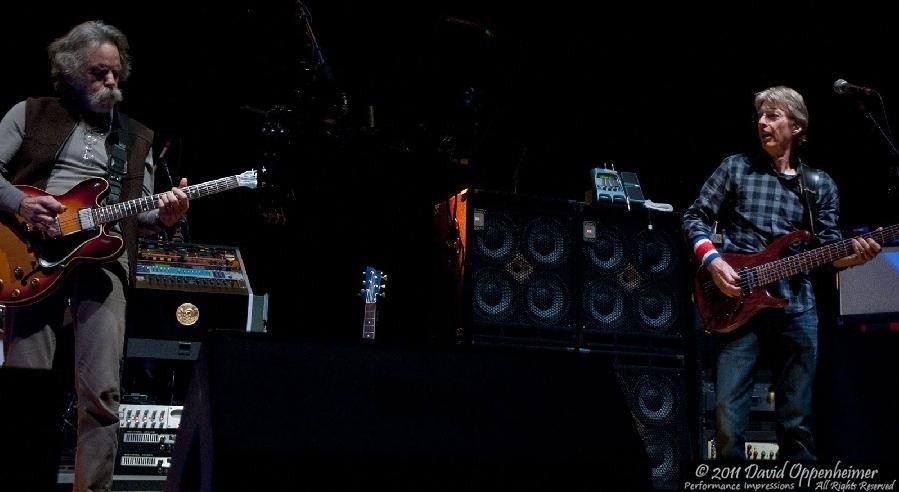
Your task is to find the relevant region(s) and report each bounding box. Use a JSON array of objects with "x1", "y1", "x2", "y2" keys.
[
  {"x1": 457, "y1": 189, "x2": 702, "y2": 492},
  {"x1": 166, "y1": 336, "x2": 652, "y2": 492}
]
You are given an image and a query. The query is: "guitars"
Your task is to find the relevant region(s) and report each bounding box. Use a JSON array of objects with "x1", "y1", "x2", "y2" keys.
[
  {"x1": 363, "y1": 267, "x2": 388, "y2": 340},
  {"x1": 695, "y1": 224, "x2": 899, "y2": 334},
  {"x1": 0, "y1": 167, "x2": 266, "y2": 308}
]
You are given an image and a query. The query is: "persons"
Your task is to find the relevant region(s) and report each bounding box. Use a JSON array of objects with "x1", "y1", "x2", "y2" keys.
[
  {"x1": 0, "y1": 21, "x2": 189, "y2": 491},
  {"x1": 683, "y1": 85, "x2": 883, "y2": 459}
]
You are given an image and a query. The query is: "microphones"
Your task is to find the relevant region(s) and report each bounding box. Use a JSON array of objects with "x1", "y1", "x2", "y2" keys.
[{"x1": 833, "y1": 79, "x2": 875, "y2": 96}]
